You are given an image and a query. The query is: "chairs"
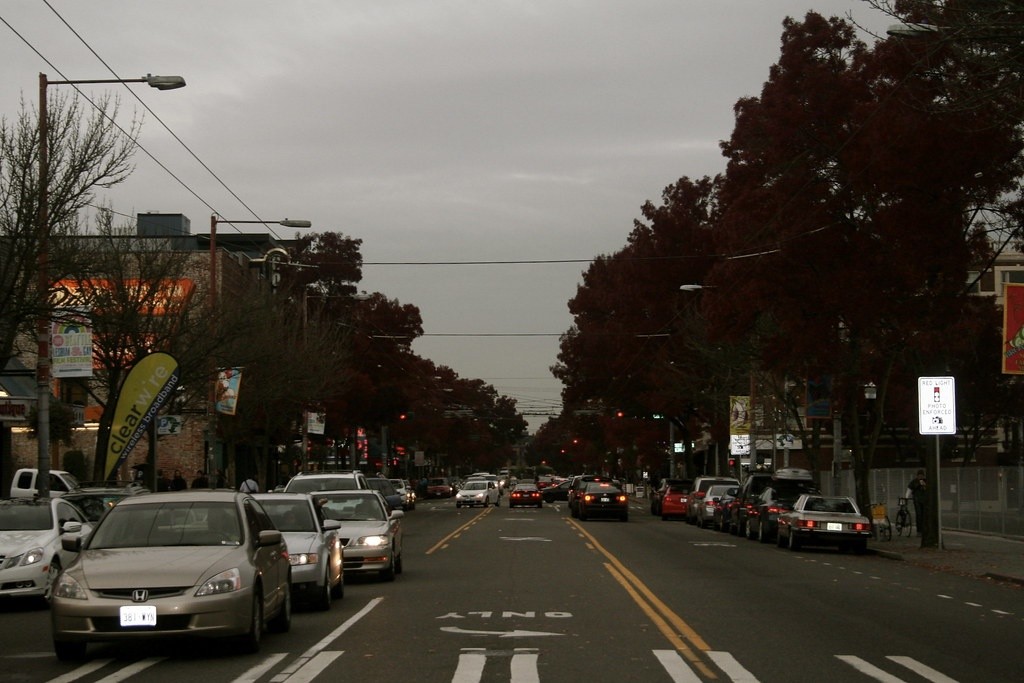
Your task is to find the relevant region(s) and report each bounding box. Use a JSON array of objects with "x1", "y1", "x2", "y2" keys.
[
  {"x1": 131, "y1": 519, "x2": 160, "y2": 538},
  {"x1": 344, "y1": 506, "x2": 354, "y2": 517}
]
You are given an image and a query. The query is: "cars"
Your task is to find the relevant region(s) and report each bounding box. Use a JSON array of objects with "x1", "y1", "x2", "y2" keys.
[{"x1": 0, "y1": 460, "x2": 881, "y2": 659}]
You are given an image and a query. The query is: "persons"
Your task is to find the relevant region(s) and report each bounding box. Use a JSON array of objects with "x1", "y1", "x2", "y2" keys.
[
  {"x1": 415, "y1": 477, "x2": 428, "y2": 498},
  {"x1": 649, "y1": 478, "x2": 660, "y2": 515},
  {"x1": 158, "y1": 464, "x2": 260, "y2": 493},
  {"x1": 904, "y1": 470, "x2": 927, "y2": 537}
]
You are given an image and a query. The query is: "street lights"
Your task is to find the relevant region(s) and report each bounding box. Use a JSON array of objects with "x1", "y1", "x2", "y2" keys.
[
  {"x1": 34, "y1": 70, "x2": 190, "y2": 503},
  {"x1": 206, "y1": 214, "x2": 315, "y2": 492}
]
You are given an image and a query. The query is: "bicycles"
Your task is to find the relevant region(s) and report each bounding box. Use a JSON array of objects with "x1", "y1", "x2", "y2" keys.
[
  {"x1": 865, "y1": 501, "x2": 892, "y2": 541},
  {"x1": 895, "y1": 497, "x2": 916, "y2": 538}
]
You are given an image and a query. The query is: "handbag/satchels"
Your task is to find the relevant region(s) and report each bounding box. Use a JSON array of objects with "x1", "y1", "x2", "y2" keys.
[{"x1": 250, "y1": 490, "x2": 256, "y2": 493}]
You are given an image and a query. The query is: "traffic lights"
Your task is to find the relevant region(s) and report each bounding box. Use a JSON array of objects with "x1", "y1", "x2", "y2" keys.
[
  {"x1": 560, "y1": 449, "x2": 566, "y2": 453},
  {"x1": 573, "y1": 439, "x2": 578, "y2": 444},
  {"x1": 400, "y1": 412, "x2": 406, "y2": 421},
  {"x1": 615, "y1": 408, "x2": 625, "y2": 421},
  {"x1": 542, "y1": 460, "x2": 548, "y2": 464},
  {"x1": 728, "y1": 457, "x2": 736, "y2": 468},
  {"x1": 651, "y1": 413, "x2": 667, "y2": 421}
]
